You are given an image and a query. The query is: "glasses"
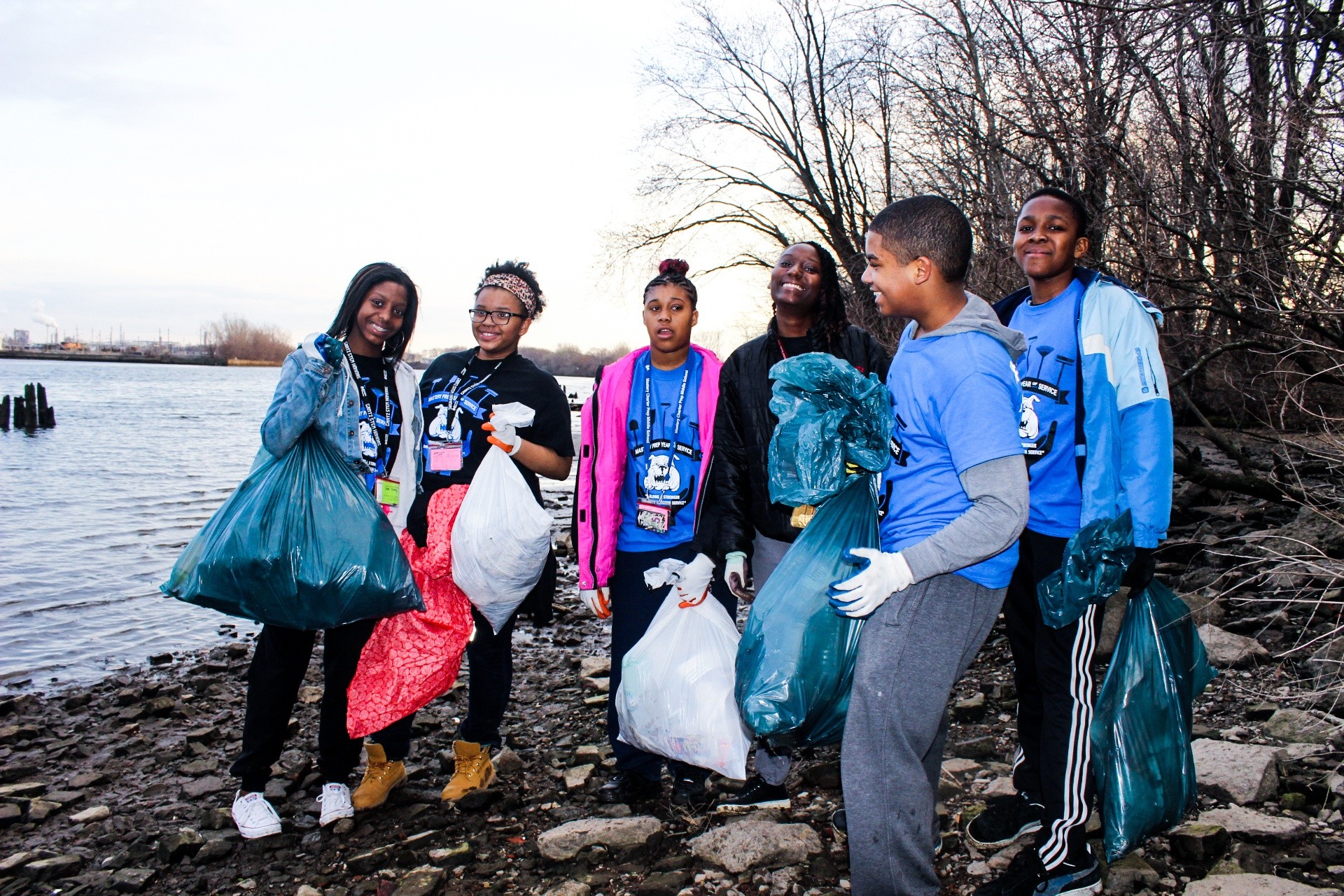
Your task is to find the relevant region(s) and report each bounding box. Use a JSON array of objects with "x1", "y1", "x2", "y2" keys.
[{"x1": 469, "y1": 308, "x2": 526, "y2": 326}]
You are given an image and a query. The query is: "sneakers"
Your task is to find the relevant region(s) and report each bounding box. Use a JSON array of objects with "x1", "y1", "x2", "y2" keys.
[
  {"x1": 350, "y1": 742, "x2": 404, "y2": 807},
  {"x1": 964, "y1": 797, "x2": 1043, "y2": 848},
  {"x1": 230, "y1": 790, "x2": 282, "y2": 838},
  {"x1": 440, "y1": 737, "x2": 495, "y2": 802},
  {"x1": 598, "y1": 771, "x2": 661, "y2": 802},
  {"x1": 967, "y1": 846, "x2": 1103, "y2": 896},
  {"x1": 315, "y1": 782, "x2": 356, "y2": 825},
  {"x1": 718, "y1": 774, "x2": 791, "y2": 812},
  {"x1": 671, "y1": 764, "x2": 704, "y2": 805}
]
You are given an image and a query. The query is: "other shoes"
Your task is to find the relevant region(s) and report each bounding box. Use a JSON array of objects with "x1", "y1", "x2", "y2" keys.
[{"x1": 830, "y1": 809, "x2": 944, "y2": 853}]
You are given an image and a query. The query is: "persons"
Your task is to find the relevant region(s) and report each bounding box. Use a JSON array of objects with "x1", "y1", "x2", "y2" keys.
[
  {"x1": 711, "y1": 241, "x2": 891, "y2": 814},
  {"x1": 571, "y1": 259, "x2": 738, "y2": 825},
  {"x1": 232, "y1": 262, "x2": 424, "y2": 839},
  {"x1": 350, "y1": 260, "x2": 576, "y2": 811},
  {"x1": 966, "y1": 187, "x2": 1173, "y2": 896},
  {"x1": 829, "y1": 198, "x2": 1029, "y2": 896}
]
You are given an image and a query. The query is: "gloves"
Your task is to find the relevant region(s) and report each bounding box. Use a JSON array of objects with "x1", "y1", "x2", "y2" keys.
[
  {"x1": 674, "y1": 553, "x2": 715, "y2": 609},
  {"x1": 826, "y1": 546, "x2": 915, "y2": 618},
  {"x1": 481, "y1": 412, "x2": 519, "y2": 457},
  {"x1": 581, "y1": 585, "x2": 610, "y2": 620},
  {"x1": 724, "y1": 552, "x2": 756, "y2": 603},
  {"x1": 301, "y1": 332, "x2": 329, "y2": 366},
  {"x1": 1117, "y1": 547, "x2": 1155, "y2": 599}
]
